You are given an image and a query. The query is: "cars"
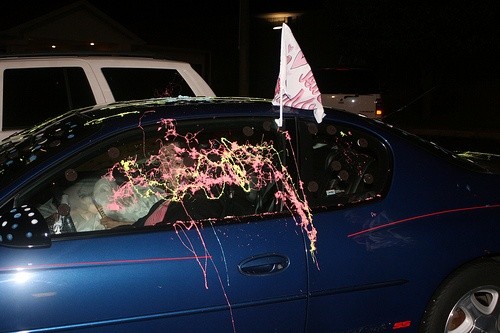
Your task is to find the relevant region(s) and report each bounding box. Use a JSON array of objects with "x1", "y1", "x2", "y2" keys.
[{"x1": 0, "y1": 96, "x2": 500, "y2": 333}]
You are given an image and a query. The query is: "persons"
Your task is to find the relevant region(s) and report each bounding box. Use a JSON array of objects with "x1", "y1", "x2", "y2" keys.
[{"x1": 100, "y1": 129, "x2": 228, "y2": 228}]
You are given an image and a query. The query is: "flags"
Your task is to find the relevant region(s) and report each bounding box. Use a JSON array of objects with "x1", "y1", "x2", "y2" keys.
[{"x1": 271, "y1": 21, "x2": 328, "y2": 125}]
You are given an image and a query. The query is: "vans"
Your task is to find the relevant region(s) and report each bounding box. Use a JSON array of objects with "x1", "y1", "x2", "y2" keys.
[{"x1": 1, "y1": 55, "x2": 220, "y2": 146}]
